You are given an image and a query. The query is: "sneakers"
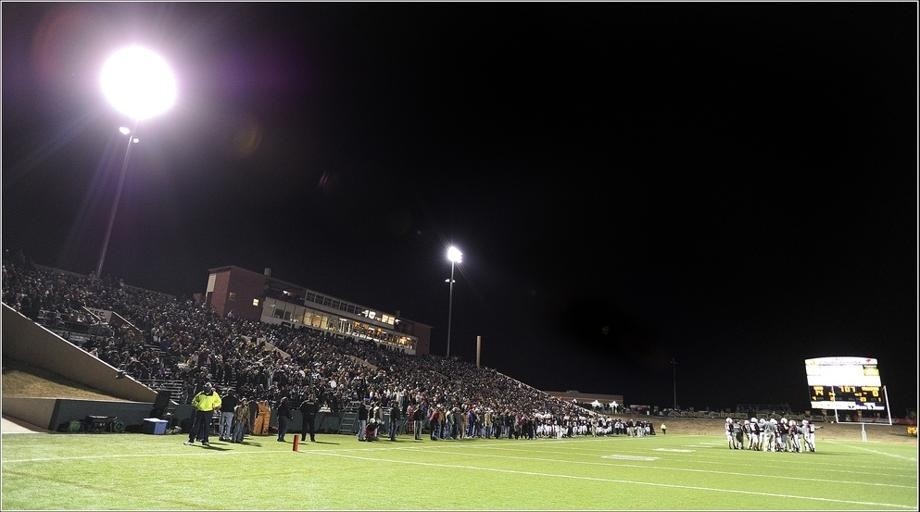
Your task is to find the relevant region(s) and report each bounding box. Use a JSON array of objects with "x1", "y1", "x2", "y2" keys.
[
  {"x1": 184, "y1": 441, "x2": 194, "y2": 445},
  {"x1": 201, "y1": 442, "x2": 211, "y2": 447}
]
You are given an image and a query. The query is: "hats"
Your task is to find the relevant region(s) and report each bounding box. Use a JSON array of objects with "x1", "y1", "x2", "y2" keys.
[{"x1": 204, "y1": 382, "x2": 212, "y2": 387}]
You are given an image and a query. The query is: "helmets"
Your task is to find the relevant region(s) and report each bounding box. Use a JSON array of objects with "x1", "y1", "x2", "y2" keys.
[{"x1": 724, "y1": 417, "x2": 809, "y2": 426}]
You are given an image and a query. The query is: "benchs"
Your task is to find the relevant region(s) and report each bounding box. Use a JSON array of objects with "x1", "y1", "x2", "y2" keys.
[{"x1": 1, "y1": 296, "x2": 246, "y2": 406}]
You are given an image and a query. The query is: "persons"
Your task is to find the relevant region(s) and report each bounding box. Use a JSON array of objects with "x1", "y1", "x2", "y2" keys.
[
  {"x1": 725, "y1": 416, "x2": 823, "y2": 454},
  {"x1": 660, "y1": 422, "x2": 667, "y2": 435}
]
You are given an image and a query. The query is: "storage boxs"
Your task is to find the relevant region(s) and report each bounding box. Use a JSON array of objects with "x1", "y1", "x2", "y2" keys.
[{"x1": 141, "y1": 417, "x2": 168, "y2": 435}]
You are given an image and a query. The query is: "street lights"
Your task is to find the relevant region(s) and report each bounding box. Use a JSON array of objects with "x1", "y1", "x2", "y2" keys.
[
  {"x1": 444, "y1": 245, "x2": 467, "y2": 358},
  {"x1": 93, "y1": 44, "x2": 180, "y2": 278}
]
